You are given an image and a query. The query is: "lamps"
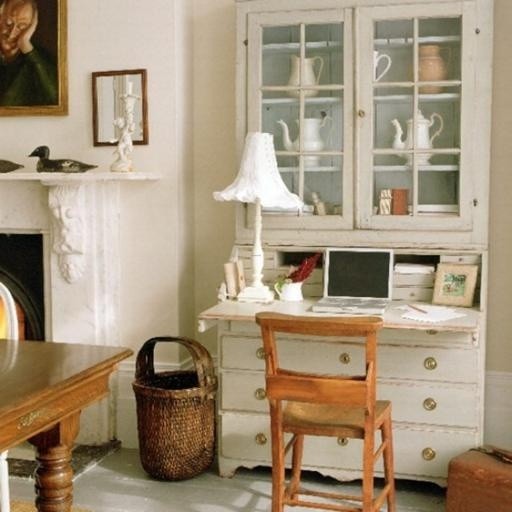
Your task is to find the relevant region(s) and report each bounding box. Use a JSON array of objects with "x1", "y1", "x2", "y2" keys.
[{"x1": 212, "y1": 132, "x2": 305, "y2": 306}]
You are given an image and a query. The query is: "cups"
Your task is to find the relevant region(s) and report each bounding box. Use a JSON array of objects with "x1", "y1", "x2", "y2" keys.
[
  {"x1": 391, "y1": 188, "x2": 409, "y2": 215},
  {"x1": 274, "y1": 281, "x2": 303, "y2": 301}
]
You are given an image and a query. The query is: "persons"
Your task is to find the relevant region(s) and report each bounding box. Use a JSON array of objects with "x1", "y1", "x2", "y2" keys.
[{"x1": 0, "y1": 1, "x2": 57, "y2": 105}]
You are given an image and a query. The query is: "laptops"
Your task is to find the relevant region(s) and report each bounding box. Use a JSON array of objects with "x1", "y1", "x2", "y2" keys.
[{"x1": 312, "y1": 246, "x2": 395, "y2": 314}]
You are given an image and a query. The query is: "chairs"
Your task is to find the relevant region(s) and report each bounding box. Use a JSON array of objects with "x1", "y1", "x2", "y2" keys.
[{"x1": 254, "y1": 311, "x2": 397, "y2": 511}]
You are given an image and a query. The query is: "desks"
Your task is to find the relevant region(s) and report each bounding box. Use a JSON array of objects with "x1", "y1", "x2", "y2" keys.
[{"x1": 0, "y1": 338, "x2": 134, "y2": 512}]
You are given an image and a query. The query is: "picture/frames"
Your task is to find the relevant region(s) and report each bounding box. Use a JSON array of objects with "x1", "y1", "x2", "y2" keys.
[
  {"x1": 0, "y1": 0, "x2": 70, "y2": 116},
  {"x1": 92, "y1": 69, "x2": 148, "y2": 148},
  {"x1": 432, "y1": 262, "x2": 479, "y2": 307}
]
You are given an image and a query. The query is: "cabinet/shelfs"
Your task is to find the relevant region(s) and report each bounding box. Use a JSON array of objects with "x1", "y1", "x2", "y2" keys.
[
  {"x1": 235, "y1": 1, "x2": 494, "y2": 248},
  {"x1": 215, "y1": 316, "x2": 487, "y2": 487}
]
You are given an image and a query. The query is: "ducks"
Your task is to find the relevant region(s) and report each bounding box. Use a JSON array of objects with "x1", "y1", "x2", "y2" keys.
[
  {"x1": 0, "y1": 160, "x2": 25, "y2": 173},
  {"x1": 27, "y1": 145, "x2": 99, "y2": 173}
]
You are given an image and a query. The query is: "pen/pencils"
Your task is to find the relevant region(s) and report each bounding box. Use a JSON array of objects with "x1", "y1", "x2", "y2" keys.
[{"x1": 407, "y1": 303, "x2": 427, "y2": 313}]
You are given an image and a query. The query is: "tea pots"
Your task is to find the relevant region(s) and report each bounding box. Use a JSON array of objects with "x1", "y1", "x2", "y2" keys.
[
  {"x1": 286, "y1": 54, "x2": 324, "y2": 98},
  {"x1": 391, "y1": 109, "x2": 443, "y2": 166},
  {"x1": 277, "y1": 116, "x2": 333, "y2": 167}
]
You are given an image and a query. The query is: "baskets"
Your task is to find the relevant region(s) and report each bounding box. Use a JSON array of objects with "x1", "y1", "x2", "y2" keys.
[{"x1": 131, "y1": 337, "x2": 218, "y2": 482}]
